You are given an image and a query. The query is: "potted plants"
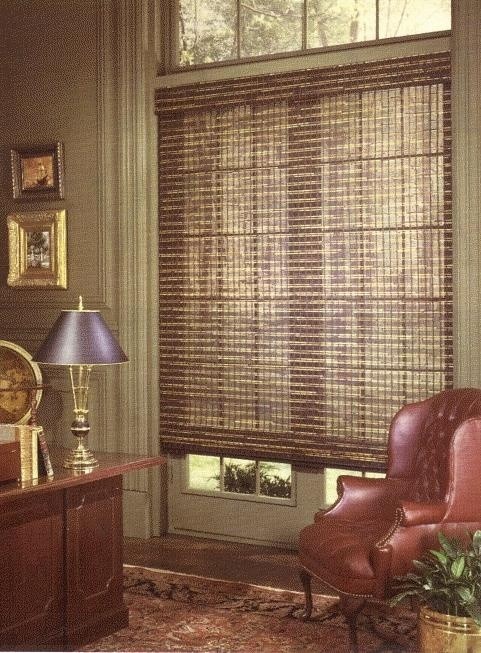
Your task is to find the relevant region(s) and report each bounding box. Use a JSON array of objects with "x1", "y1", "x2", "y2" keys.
[{"x1": 384, "y1": 530, "x2": 480, "y2": 651}]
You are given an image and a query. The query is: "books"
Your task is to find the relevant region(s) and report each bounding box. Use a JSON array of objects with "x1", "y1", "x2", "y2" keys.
[{"x1": 0, "y1": 424, "x2": 54, "y2": 482}]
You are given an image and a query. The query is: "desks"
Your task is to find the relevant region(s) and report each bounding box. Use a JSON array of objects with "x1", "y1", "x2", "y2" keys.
[{"x1": 1, "y1": 452, "x2": 164, "y2": 651}]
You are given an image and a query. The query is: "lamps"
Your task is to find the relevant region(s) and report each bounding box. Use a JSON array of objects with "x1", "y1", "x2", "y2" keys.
[{"x1": 31, "y1": 293, "x2": 130, "y2": 471}]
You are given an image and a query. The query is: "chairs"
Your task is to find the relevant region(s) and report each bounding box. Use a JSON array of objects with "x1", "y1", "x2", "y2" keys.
[{"x1": 298, "y1": 387, "x2": 480, "y2": 652}]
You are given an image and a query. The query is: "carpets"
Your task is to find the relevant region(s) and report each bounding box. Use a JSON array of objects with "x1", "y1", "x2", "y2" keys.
[{"x1": 83, "y1": 564, "x2": 419, "y2": 653}]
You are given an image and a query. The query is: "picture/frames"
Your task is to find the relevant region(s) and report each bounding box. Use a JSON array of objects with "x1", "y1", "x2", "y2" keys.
[{"x1": 4, "y1": 140, "x2": 71, "y2": 288}]
